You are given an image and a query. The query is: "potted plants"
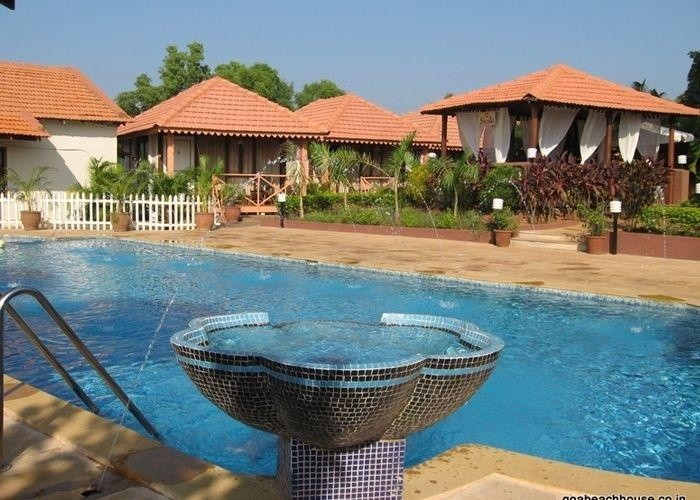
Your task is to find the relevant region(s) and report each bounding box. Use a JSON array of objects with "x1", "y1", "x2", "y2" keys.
[
  {"x1": 0, "y1": 162, "x2": 58, "y2": 230},
  {"x1": 178, "y1": 154, "x2": 246, "y2": 232},
  {"x1": 579, "y1": 202, "x2": 608, "y2": 255},
  {"x1": 90, "y1": 159, "x2": 152, "y2": 231},
  {"x1": 494, "y1": 208, "x2": 515, "y2": 246}
]
